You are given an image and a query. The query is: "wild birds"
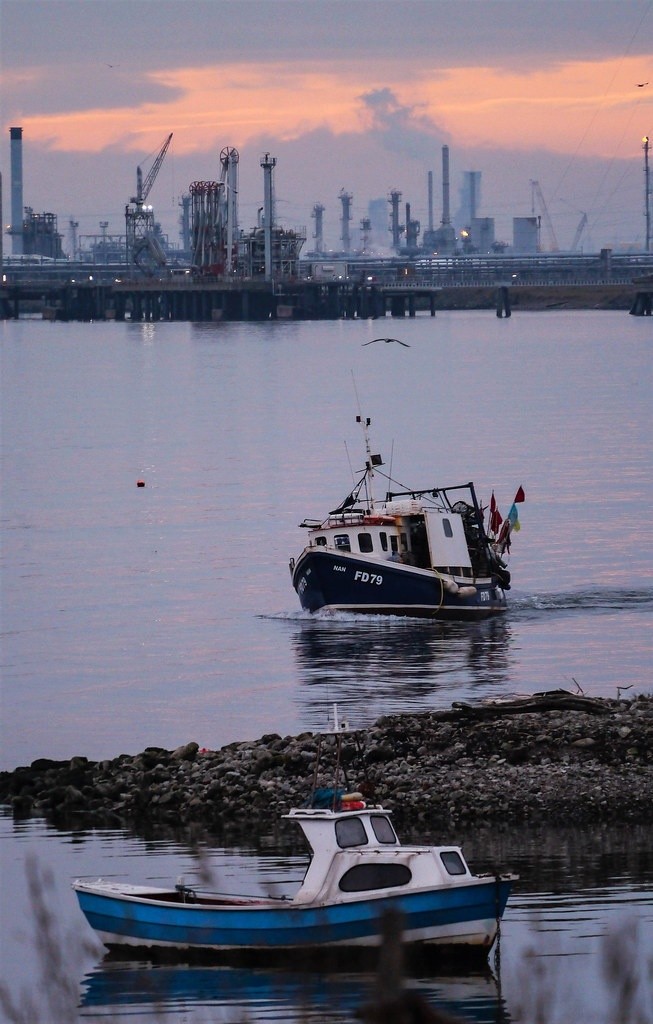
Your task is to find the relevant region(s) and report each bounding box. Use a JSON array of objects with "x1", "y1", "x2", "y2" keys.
[
  {"x1": 634, "y1": 82, "x2": 649, "y2": 88},
  {"x1": 105, "y1": 62, "x2": 121, "y2": 68},
  {"x1": 361, "y1": 337, "x2": 411, "y2": 348}
]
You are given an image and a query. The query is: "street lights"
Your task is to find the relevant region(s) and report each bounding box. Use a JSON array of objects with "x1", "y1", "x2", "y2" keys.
[{"x1": 641, "y1": 136, "x2": 649, "y2": 249}]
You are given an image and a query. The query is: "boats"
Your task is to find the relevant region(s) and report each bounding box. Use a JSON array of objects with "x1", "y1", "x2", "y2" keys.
[
  {"x1": 65, "y1": 781, "x2": 517, "y2": 972},
  {"x1": 286, "y1": 461, "x2": 526, "y2": 614}
]
